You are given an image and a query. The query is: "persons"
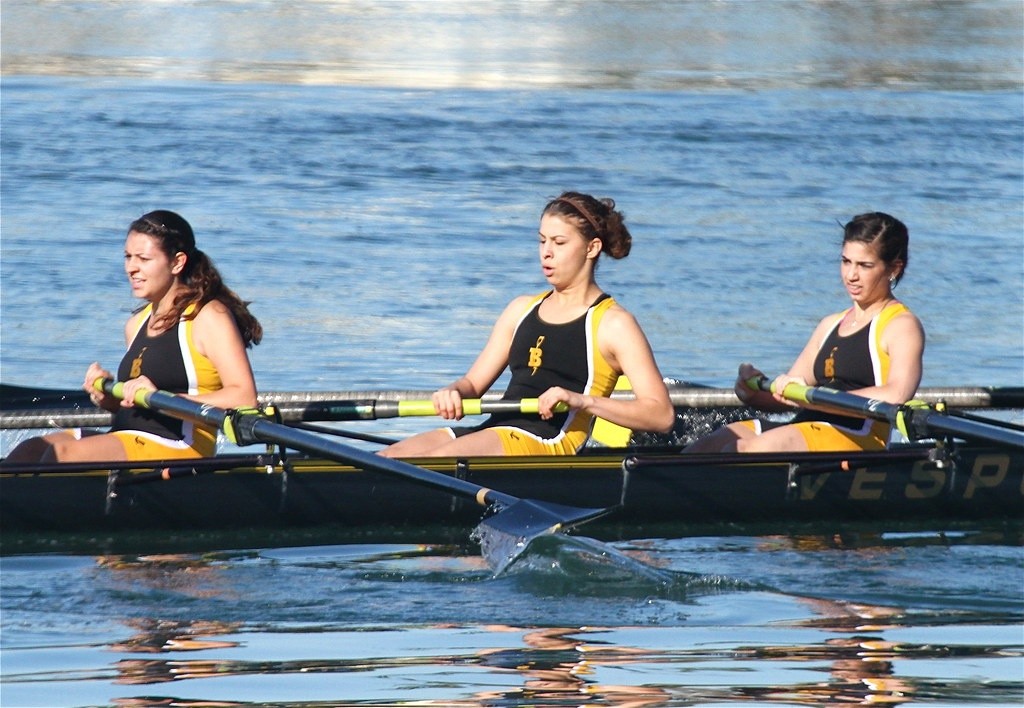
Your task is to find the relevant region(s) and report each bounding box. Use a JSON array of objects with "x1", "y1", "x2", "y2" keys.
[
  {"x1": 691, "y1": 211, "x2": 924, "y2": 452},
  {"x1": 371, "y1": 190, "x2": 678, "y2": 460},
  {"x1": 0, "y1": 210, "x2": 262, "y2": 466}
]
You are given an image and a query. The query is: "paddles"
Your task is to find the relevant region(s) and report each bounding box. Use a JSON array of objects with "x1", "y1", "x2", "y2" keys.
[
  {"x1": 744, "y1": 375, "x2": 1024, "y2": 448},
  {"x1": 86, "y1": 376, "x2": 621, "y2": 538},
  {"x1": 0, "y1": 382, "x2": 571, "y2": 416}
]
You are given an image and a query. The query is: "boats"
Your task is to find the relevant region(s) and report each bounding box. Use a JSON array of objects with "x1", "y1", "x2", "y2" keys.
[{"x1": 0, "y1": 447, "x2": 1024, "y2": 553}]
